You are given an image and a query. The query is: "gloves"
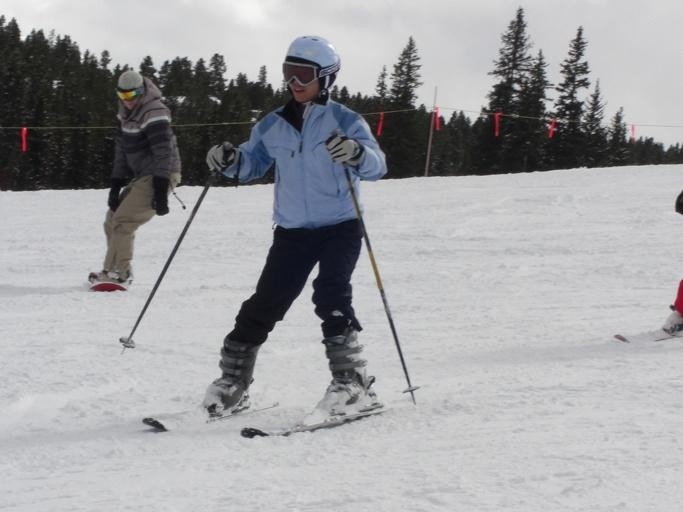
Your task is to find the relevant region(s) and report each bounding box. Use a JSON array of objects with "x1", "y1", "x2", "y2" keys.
[
  {"x1": 325, "y1": 133, "x2": 364, "y2": 164},
  {"x1": 153, "y1": 176, "x2": 169, "y2": 215},
  {"x1": 206, "y1": 140, "x2": 240, "y2": 173},
  {"x1": 108, "y1": 177, "x2": 121, "y2": 210}
]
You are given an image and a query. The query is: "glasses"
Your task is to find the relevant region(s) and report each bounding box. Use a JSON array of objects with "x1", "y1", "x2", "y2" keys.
[
  {"x1": 115, "y1": 88, "x2": 143, "y2": 101},
  {"x1": 283, "y1": 62, "x2": 318, "y2": 86}
]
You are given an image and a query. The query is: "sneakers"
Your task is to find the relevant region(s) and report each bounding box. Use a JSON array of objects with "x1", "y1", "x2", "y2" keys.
[
  {"x1": 663, "y1": 311, "x2": 683, "y2": 333},
  {"x1": 89, "y1": 270, "x2": 130, "y2": 284}
]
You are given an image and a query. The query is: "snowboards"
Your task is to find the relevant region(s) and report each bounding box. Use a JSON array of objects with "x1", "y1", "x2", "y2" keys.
[{"x1": 92, "y1": 268, "x2": 133, "y2": 292}]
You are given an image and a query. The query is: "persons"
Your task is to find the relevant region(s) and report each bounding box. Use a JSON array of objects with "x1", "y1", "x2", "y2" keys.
[
  {"x1": 200, "y1": 34, "x2": 388, "y2": 416},
  {"x1": 87, "y1": 69, "x2": 182, "y2": 286},
  {"x1": 662, "y1": 189, "x2": 683, "y2": 334}
]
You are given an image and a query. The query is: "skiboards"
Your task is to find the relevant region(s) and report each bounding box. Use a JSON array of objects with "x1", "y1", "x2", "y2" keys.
[
  {"x1": 614, "y1": 309, "x2": 683, "y2": 344},
  {"x1": 141, "y1": 375, "x2": 429, "y2": 438}
]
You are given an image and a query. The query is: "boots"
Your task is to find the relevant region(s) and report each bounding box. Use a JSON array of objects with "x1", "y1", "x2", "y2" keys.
[
  {"x1": 310, "y1": 328, "x2": 380, "y2": 415},
  {"x1": 204, "y1": 329, "x2": 262, "y2": 414}
]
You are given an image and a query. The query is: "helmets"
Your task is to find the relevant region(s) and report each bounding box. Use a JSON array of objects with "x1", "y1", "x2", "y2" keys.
[
  {"x1": 117, "y1": 71, "x2": 144, "y2": 90},
  {"x1": 286, "y1": 35, "x2": 340, "y2": 72}
]
